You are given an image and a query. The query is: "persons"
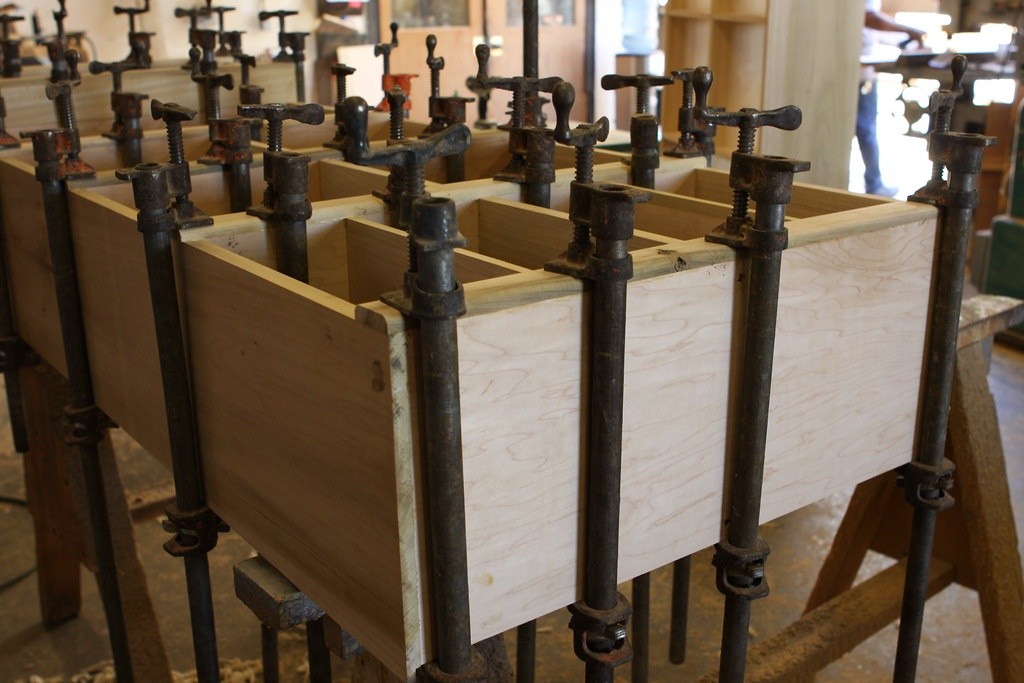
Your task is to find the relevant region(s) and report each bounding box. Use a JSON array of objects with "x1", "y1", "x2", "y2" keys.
[{"x1": 855, "y1": 0, "x2": 927, "y2": 198}]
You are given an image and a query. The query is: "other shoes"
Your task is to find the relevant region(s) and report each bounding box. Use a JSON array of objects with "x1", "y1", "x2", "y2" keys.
[{"x1": 874, "y1": 187, "x2": 898, "y2": 197}]
